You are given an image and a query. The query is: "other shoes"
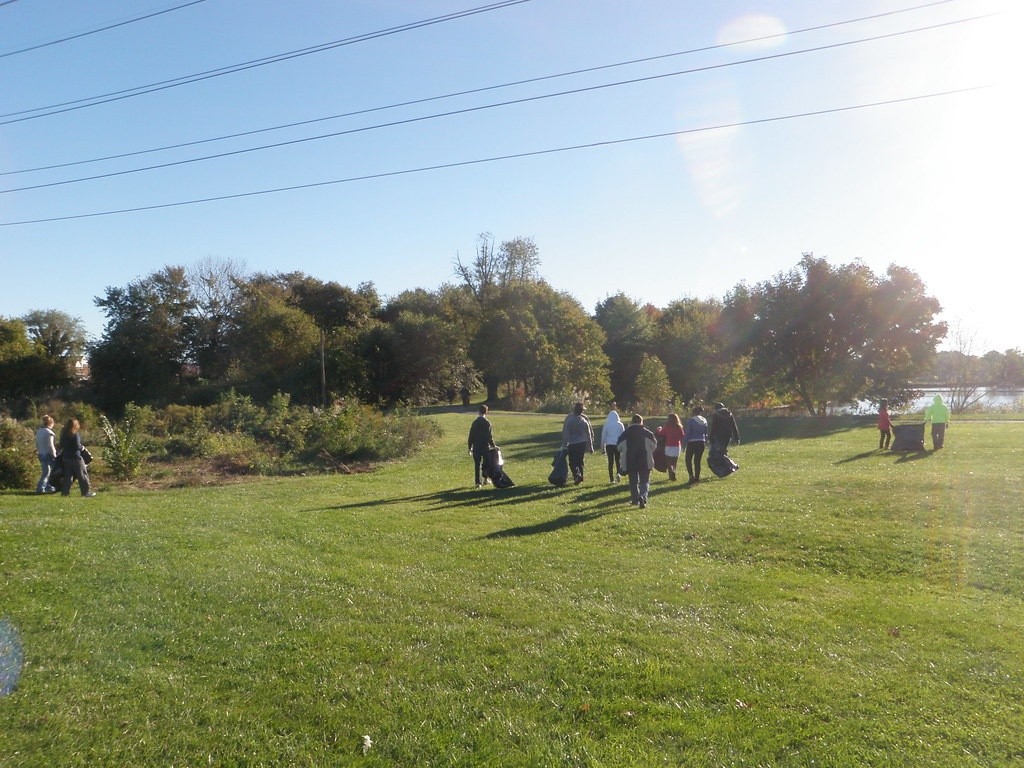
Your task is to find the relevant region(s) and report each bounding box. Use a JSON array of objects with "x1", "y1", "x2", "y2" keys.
[
  {"x1": 36, "y1": 488, "x2": 54, "y2": 494},
  {"x1": 609, "y1": 473, "x2": 621, "y2": 485},
  {"x1": 476, "y1": 480, "x2": 490, "y2": 490},
  {"x1": 573, "y1": 476, "x2": 584, "y2": 485},
  {"x1": 687, "y1": 476, "x2": 700, "y2": 484},
  {"x1": 629, "y1": 499, "x2": 646, "y2": 509},
  {"x1": 934, "y1": 445, "x2": 945, "y2": 449},
  {"x1": 880, "y1": 447, "x2": 892, "y2": 451},
  {"x1": 83, "y1": 492, "x2": 96, "y2": 497},
  {"x1": 669, "y1": 471, "x2": 676, "y2": 480},
  {"x1": 61, "y1": 492, "x2": 72, "y2": 497}
]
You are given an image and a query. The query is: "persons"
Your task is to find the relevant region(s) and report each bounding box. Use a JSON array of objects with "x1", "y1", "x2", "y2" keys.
[
  {"x1": 711, "y1": 402, "x2": 741, "y2": 475},
  {"x1": 656, "y1": 414, "x2": 685, "y2": 480},
  {"x1": 561, "y1": 403, "x2": 594, "y2": 483},
  {"x1": 600, "y1": 409, "x2": 626, "y2": 483},
  {"x1": 924, "y1": 395, "x2": 950, "y2": 451},
  {"x1": 877, "y1": 398, "x2": 895, "y2": 451},
  {"x1": 60, "y1": 418, "x2": 97, "y2": 498},
  {"x1": 617, "y1": 414, "x2": 658, "y2": 507},
  {"x1": 35, "y1": 414, "x2": 58, "y2": 495},
  {"x1": 680, "y1": 405, "x2": 709, "y2": 483},
  {"x1": 467, "y1": 404, "x2": 500, "y2": 490}
]
